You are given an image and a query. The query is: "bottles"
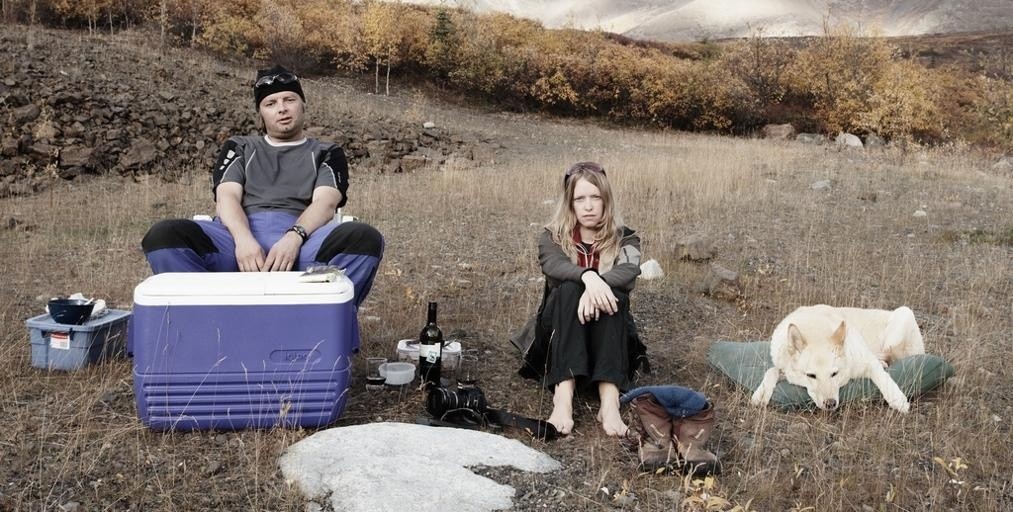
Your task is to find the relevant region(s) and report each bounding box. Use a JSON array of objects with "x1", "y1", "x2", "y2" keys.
[{"x1": 419, "y1": 301, "x2": 443, "y2": 391}]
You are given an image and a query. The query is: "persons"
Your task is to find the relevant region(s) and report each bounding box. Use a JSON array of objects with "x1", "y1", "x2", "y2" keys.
[
  {"x1": 509, "y1": 162, "x2": 647, "y2": 436},
  {"x1": 141, "y1": 66, "x2": 385, "y2": 311}
]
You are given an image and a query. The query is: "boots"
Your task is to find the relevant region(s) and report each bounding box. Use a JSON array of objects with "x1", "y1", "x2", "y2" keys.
[{"x1": 630, "y1": 394, "x2": 723, "y2": 476}]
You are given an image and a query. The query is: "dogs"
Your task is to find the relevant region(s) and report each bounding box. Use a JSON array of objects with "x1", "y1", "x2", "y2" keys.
[{"x1": 749, "y1": 304, "x2": 926, "y2": 415}]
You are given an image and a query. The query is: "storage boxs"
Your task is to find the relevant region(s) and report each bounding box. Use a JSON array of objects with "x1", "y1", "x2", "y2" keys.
[
  {"x1": 25, "y1": 305, "x2": 133, "y2": 371},
  {"x1": 127, "y1": 270, "x2": 360, "y2": 431}
]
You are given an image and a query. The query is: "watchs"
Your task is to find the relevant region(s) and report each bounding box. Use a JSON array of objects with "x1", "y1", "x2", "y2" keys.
[{"x1": 286, "y1": 224, "x2": 307, "y2": 241}]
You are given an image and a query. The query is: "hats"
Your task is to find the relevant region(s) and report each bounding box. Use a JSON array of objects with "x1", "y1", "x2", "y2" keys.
[{"x1": 254, "y1": 64, "x2": 306, "y2": 108}]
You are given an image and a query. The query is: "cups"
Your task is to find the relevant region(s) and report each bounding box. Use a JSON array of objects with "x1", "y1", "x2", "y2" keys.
[
  {"x1": 456, "y1": 355, "x2": 480, "y2": 387},
  {"x1": 365, "y1": 357, "x2": 389, "y2": 385}
]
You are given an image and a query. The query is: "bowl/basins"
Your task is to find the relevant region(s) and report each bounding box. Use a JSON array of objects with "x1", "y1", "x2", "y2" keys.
[
  {"x1": 378, "y1": 361, "x2": 416, "y2": 386},
  {"x1": 47, "y1": 299, "x2": 95, "y2": 325}
]
